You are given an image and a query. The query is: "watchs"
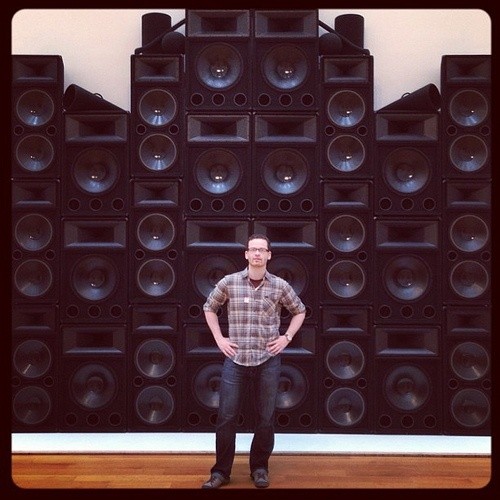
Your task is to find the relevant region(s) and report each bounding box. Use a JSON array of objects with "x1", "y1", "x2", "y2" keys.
[{"x1": 284, "y1": 333, "x2": 292, "y2": 341}]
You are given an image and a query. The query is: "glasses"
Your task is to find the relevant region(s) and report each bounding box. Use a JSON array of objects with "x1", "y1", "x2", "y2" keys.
[{"x1": 247, "y1": 248, "x2": 269, "y2": 253}]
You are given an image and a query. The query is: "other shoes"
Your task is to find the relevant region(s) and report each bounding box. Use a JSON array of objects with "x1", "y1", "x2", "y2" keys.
[
  {"x1": 253, "y1": 470, "x2": 271, "y2": 487},
  {"x1": 202, "y1": 472, "x2": 231, "y2": 488}
]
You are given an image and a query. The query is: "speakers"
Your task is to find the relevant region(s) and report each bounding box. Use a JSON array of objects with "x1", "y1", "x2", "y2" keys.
[{"x1": 11, "y1": 9, "x2": 493, "y2": 434}]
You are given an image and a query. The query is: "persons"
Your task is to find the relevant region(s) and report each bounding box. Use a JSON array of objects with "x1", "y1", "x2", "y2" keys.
[{"x1": 202, "y1": 234, "x2": 306, "y2": 488}]
